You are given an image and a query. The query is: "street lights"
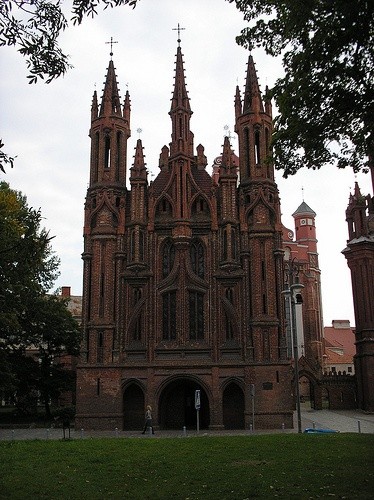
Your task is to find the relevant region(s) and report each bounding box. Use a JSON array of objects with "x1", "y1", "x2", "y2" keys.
[{"x1": 282, "y1": 283, "x2": 306, "y2": 434}]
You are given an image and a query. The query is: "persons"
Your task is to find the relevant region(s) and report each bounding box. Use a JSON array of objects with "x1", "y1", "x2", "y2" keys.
[{"x1": 141, "y1": 406, "x2": 156, "y2": 434}]
